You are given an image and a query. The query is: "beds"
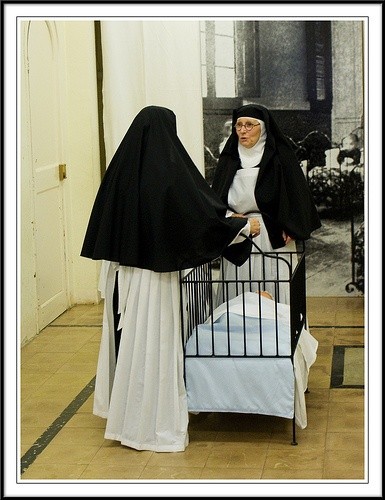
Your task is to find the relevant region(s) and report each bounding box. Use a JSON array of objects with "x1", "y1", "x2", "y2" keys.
[{"x1": 179, "y1": 249, "x2": 318, "y2": 448}]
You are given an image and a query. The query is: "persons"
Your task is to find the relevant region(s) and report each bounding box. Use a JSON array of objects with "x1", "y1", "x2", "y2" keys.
[
  {"x1": 254, "y1": 288, "x2": 272, "y2": 305},
  {"x1": 337, "y1": 134, "x2": 362, "y2": 181},
  {"x1": 205, "y1": 104, "x2": 321, "y2": 309},
  {"x1": 77, "y1": 106, "x2": 262, "y2": 455}
]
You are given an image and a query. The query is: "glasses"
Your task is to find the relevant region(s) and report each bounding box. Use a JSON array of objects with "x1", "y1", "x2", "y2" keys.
[{"x1": 233, "y1": 122, "x2": 260, "y2": 131}]
